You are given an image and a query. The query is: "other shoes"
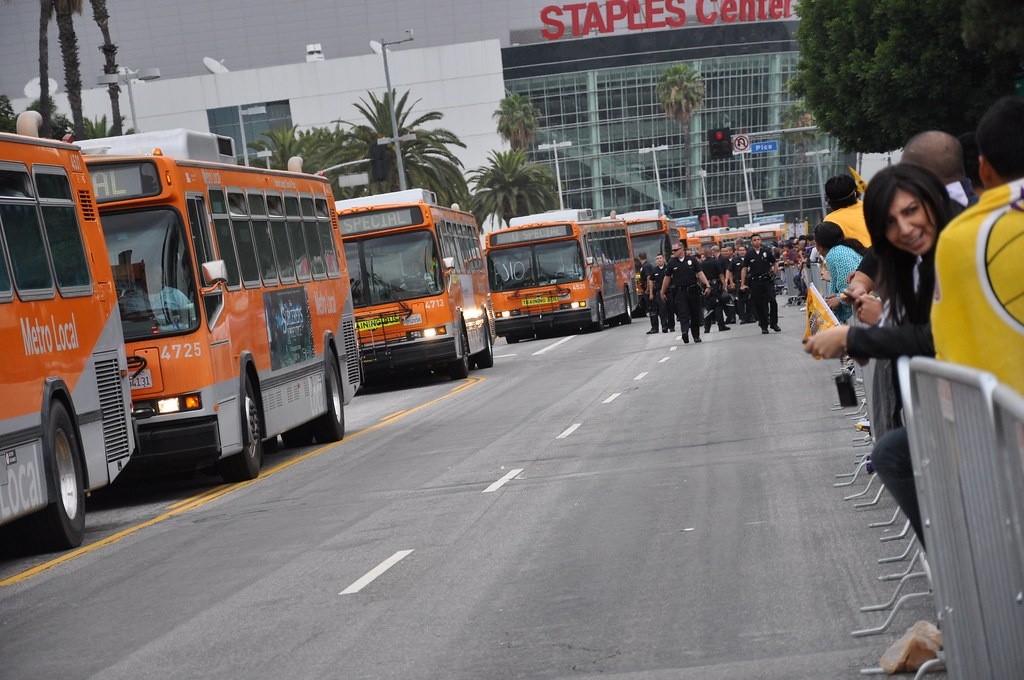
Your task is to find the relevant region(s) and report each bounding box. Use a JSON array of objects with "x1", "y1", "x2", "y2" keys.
[
  {"x1": 704, "y1": 328, "x2": 710, "y2": 333},
  {"x1": 694, "y1": 336, "x2": 701, "y2": 342},
  {"x1": 724, "y1": 316, "x2": 758, "y2": 324},
  {"x1": 682, "y1": 336, "x2": 690, "y2": 343},
  {"x1": 761, "y1": 327, "x2": 769, "y2": 334},
  {"x1": 719, "y1": 326, "x2": 730, "y2": 331},
  {"x1": 663, "y1": 328, "x2": 669, "y2": 333},
  {"x1": 770, "y1": 324, "x2": 781, "y2": 332},
  {"x1": 647, "y1": 328, "x2": 660, "y2": 334},
  {"x1": 670, "y1": 328, "x2": 675, "y2": 332}
]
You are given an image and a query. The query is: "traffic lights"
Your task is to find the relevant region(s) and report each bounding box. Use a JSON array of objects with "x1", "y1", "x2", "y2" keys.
[{"x1": 708, "y1": 127, "x2": 732, "y2": 161}]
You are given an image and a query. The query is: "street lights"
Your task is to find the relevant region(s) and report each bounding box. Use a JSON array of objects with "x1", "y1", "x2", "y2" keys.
[
  {"x1": 376, "y1": 134, "x2": 417, "y2": 192},
  {"x1": 95, "y1": 66, "x2": 161, "y2": 134},
  {"x1": 638, "y1": 144, "x2": 669, "y2": 217},
  {"x1": 257, "y1": 146, "x2": 272, "y2": 169},
  {"x1": 731, "y1": 149, "x2": 753, "y2": 224},
  {"x1": 699, "y1": 169, "x2": 710, "y2": 229},
  {"x1": 539, "y1": 139, "x2": 571, "y2": 210},
  {"x1": 804, "y1": 149, "x2": 831, "y2": 221},
  {"x1": 236, "y1": 103, "x2": 267, "y2": 166}
]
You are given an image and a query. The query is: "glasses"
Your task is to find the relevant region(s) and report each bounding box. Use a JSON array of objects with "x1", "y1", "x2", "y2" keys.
[{"x1": 672, "y1": 248, "x2": 681, "y2": 252}]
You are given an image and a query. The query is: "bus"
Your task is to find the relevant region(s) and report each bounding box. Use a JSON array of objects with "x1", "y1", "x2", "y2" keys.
[
  {"x1": 600, "y1": 210, "x2": 786, "y2": 314},
  {"x1": 487, "y1": 209, "x2": 639, "y2": 346},
  {"x1": 333, "y1": 187, "x2": 496, "y2": 392},
  {"x1": 68, "y1": 127, "x2": 363, "y2": 484},
  {"x1": 0, "y1": 133, "x2": 139, "y2": 551}
]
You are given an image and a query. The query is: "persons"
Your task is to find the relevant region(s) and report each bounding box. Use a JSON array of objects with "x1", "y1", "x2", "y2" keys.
[
  {"x1": 556, "y1": 257, "x2": 582, "y2": 276},
  {"x1": 813, "y1": 221, "x2": 868, "y2": 324},
  {"x1": 125, "y1": 263, "x2": 191, "y2": 319},
  {"x1": 639, "y1": 234, "x2": 781, "y2": 343},
  {"x1": 804, "y1": 164, "x2": 956, "y2": 553},
  {"x1": 843, "y1": 131, "x2": 984, "y2": 324},
  {"x1": 930, "y1": 97, "x2": 1024, "y2": 397},
  {"x1": 768, "y1": 234, "x2": 820, "y2": 265},
  {"x1": 823, "y1": 175, "x2": 872, "y2": 248},
  {"x1": 400, "y1": 262, "x2": 432, "y2": 288}
]
